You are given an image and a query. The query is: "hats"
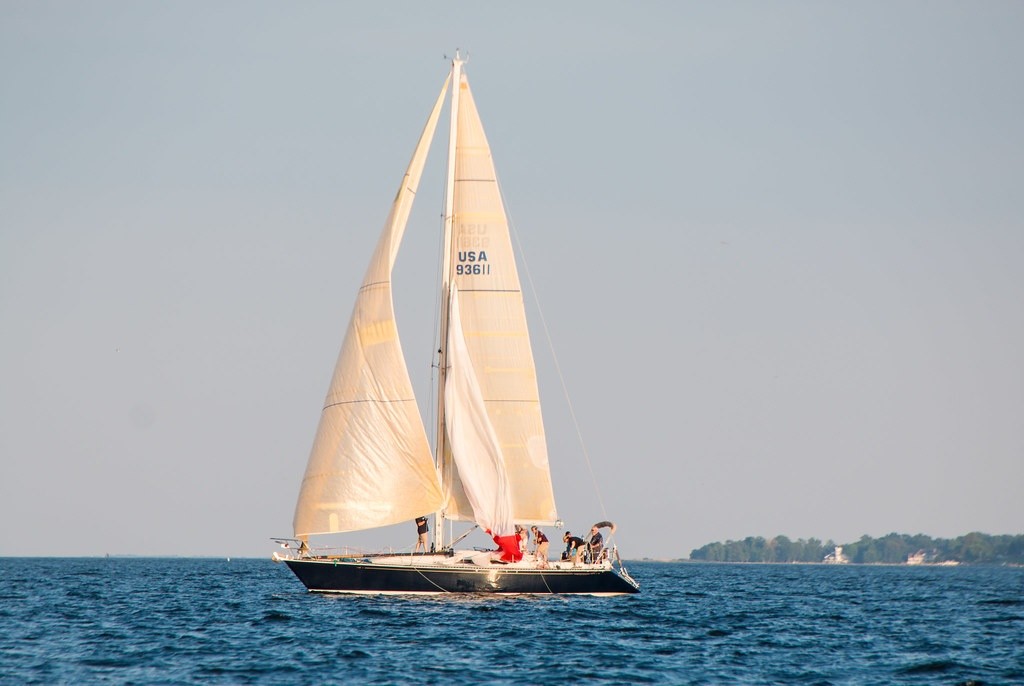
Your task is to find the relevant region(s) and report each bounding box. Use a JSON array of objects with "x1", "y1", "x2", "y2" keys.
[{"x1": 563, "y1": 536, "x2": 569, "y2": 542}]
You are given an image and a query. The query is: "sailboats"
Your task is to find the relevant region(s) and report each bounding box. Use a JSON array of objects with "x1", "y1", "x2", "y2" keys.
[{"x1": 270, "y1": 44, "x2": 644, "y2": 594}]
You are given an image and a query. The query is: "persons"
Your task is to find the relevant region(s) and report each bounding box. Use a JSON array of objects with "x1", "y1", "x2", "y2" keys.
[
  {"x1": 515, "y1": 525, "x2": 530, "y2": 548},
  {"x1": 531, "y1": 526, "x2": 549, "y2": 562},
  {"x1": 565, "y1": 532, "x2": 574, "y2": 557},
  {"x1": 587, "y1": 527, "x2": 603, "y2": 564},
  {"x1": 563, "y1": 536, "x2": 585, "y2": 563},
  {"x1": 415, "y1": 516, "x2": 429, "y2": 552}
]
